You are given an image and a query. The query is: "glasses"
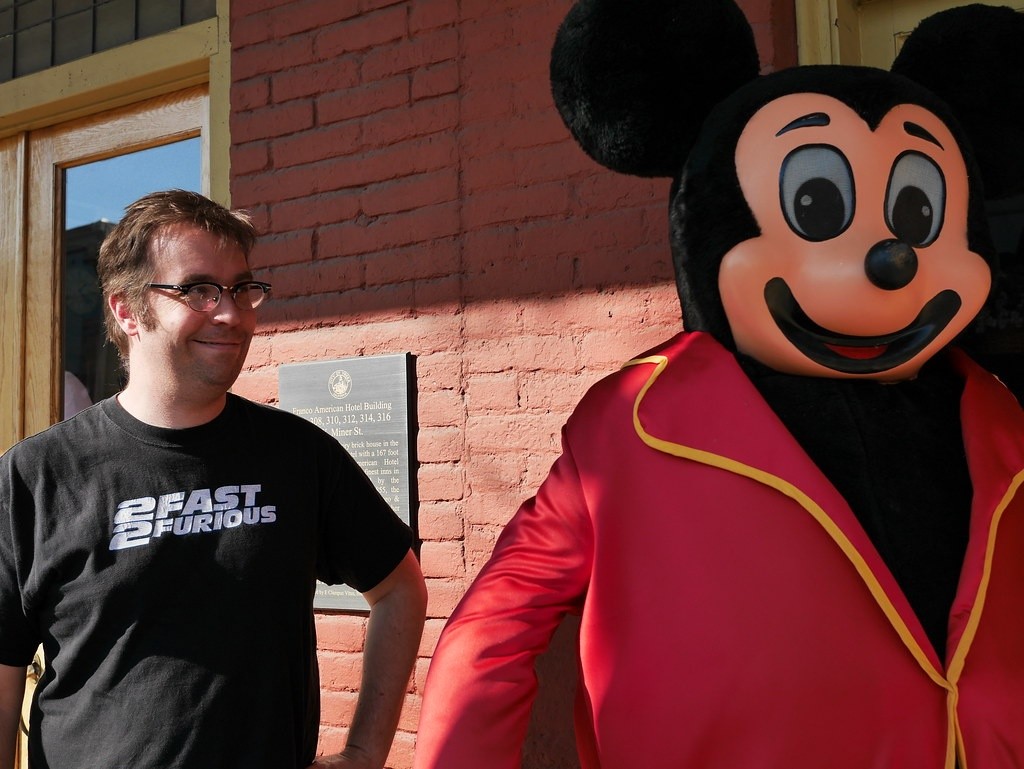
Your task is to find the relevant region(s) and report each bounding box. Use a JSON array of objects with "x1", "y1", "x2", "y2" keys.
[{"x1": 148, "y1": 280, "x2": 272, "y2": 312}]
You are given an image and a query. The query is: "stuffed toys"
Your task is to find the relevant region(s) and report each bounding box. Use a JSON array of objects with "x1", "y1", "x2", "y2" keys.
[{"x1": 412, "y1": 1, "x2": 1024, "y2": 769}]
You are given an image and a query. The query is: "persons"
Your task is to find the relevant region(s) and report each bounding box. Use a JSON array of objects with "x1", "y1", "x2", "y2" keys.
[{"x1": 0, "y1": 188, "x2": 428, "y2": 769}]
